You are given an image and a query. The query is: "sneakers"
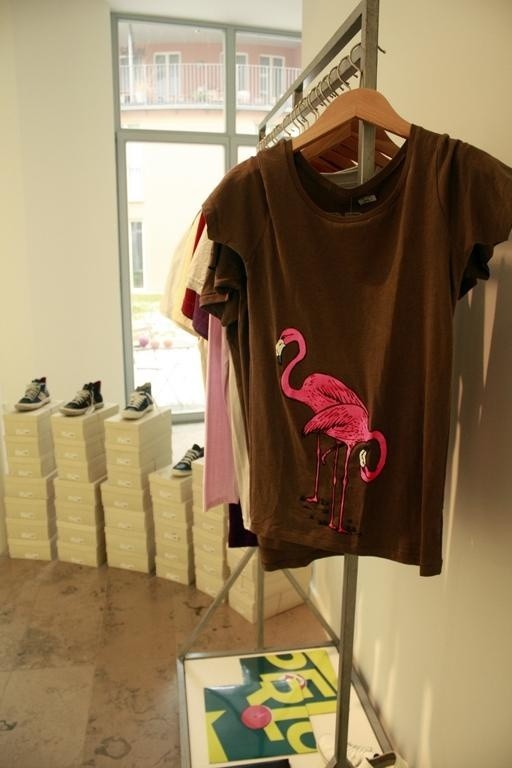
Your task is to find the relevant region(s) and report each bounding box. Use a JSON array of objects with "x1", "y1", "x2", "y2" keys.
[
  {"x1": 60, "y1": 381, "x2": 104, "y2": 416},
  {"x1": 14, "y1": 377, "x2": 51, "y2": 411},
  {"x1": 121, "y1": 382, "x2": 154, "y2": 419},
  {"x1": 172, "y1": 443, "x2": 204, "y2": 476}
]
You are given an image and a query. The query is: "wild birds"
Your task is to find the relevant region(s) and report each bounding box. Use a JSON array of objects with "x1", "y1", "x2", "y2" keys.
[
  {"x1": 299, "y1": 404, "x2": 388, "y2": 532},
  {"x1": 274, "y1": 328, "x2": 369, "y2": 504}
]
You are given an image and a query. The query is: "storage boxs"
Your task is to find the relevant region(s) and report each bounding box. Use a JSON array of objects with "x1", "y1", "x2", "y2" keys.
[{"x1": 0, "y1": 400, "x2": 319, "y2": 626}]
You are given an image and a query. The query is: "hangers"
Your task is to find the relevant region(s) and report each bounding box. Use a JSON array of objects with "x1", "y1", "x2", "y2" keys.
[{"x1": 255, "y1": 41, "x2": 414, "y2": 172}]
